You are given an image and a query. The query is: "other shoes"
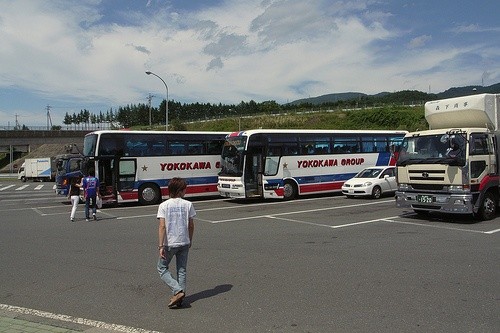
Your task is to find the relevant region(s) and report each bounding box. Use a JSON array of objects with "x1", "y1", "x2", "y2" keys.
[
  {"x1": 70, "y1": 217, "x2": 75, "y2": 222},
  {"x1": 92, "y1": 213, "x2": 97, "y2": 220},
  {"x1": 175, "y1": 299, "x2": 184, "y2": 306},
  {"x1": 167, "y1": 292, "x2": 186, "y2": 307},
  {"x1": 85, "y1": 219, "x2": 90, "y2": 222}
]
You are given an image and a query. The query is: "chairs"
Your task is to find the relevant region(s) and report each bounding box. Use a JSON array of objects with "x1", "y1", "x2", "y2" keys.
[
  {"x1": 373, "y1": 147, "x2": 377, "y2": 152},
  {"x1": 304, "y1": 145, "x2": 360, "y2": 155},
  {"x1": 386, "y1": 145, "x2": 401, "y2": 152}
]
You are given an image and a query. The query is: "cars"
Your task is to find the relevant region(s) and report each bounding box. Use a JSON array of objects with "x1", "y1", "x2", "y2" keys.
[{"x1": 340, "y1": 165, "x2": 397, "y2": 199}]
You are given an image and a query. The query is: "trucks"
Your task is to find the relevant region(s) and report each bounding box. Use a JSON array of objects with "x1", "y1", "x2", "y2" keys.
[
  {"x1": 17, "y1": 156, "x2": 58, "y2": 182},
  {"x1": 54, "y1": 153, "x2": 84, "y2": 196}
]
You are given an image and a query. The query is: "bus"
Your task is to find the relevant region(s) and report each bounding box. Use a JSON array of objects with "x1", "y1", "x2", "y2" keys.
[
  {"x1": 80, "y1": 129, "x2": 239, "y2": 208},
  {"x1": 216, "y1": 129, "x2": 415, "y2": 203}
]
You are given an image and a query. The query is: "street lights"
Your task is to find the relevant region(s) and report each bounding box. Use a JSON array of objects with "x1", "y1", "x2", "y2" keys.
[{"x1": 144, "y1": 70, "x2": 169, "y2": 131}]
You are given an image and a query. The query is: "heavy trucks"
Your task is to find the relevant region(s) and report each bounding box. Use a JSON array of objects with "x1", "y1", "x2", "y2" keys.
[{"x1": 396, "y1": 92, "x2": 500, "y2": 221}]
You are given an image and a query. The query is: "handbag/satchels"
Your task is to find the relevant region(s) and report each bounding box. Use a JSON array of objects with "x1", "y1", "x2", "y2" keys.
[
  {"x1": 67, "y1": 194, "x2": 71, "y2": 201},
  {"x1": 95, "y1": 195, "x2": 102, "y2": 208}
]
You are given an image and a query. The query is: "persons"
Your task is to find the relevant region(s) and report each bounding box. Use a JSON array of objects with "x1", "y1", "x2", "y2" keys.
[
  {"x1": 440, "y1": 138, "x2": 461, "y2": 158},
  {"x1": 68, "y1": 170, "x2": 101, "y2": 222},
  {"x1": 157, "y1": 177, "x2": 197, "y2": 309},
  {"x1": 307, "y1": 145, "x2": 360, "y2": 154}
]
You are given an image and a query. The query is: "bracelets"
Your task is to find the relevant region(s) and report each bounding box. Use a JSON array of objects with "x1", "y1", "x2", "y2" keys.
[{"x1": 158, "y1": 245, "x2": 163, "y2": 249}]
